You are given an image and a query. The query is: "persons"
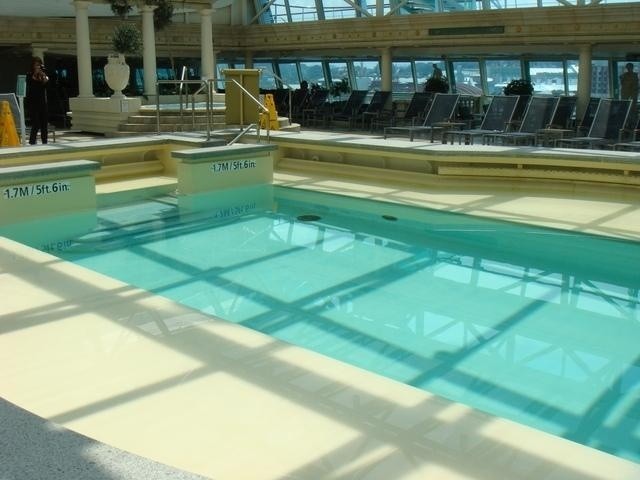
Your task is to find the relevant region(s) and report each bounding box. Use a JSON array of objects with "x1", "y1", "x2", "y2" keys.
[
  {"x1": 619, "y1": 62, "x2": 640, "y2": 112},
  {"x1": 432, "y1": 63, "x2": 442, "y2": 80},
  {"x1": 25, "y1": 55, "x2": 50, "y2": 144}
]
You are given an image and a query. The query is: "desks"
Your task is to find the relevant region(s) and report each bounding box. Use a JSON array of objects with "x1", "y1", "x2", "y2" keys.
[
  {"x1": 535, "y1": 128, "x2": 574, "y2": 147},
  {"x1": 430, "y1": 122, "x2": 465, "y2": 144}
]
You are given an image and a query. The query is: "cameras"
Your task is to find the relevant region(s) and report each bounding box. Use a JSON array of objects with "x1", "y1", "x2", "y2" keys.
[{"x1": 38, "y1": 64, "x2": 45, "y2": 72}]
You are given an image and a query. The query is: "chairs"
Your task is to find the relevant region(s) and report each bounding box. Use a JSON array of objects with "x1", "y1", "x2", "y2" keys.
[
  {"x1": 259, "y1": 89, "x2": 330, "y2": 128},
  {"x1": 554, "y1": 97, "x2": 634, "y2": 148},
  {"x1": 352, "y1": 90, "x2": 393, "y2": 132},
  {"x1": 441, "y1": 94, "x2": 521, "y2": 144},
  {"x1": 615, "y1": 139, "x2": 639, "y2": 150},
  {"x1": 0, "y1": 93, "x2": 56, "y2": 146},
  {"x1": 483, "y1": 95, "x2": 563, "y2": 147},
  {"x1": 328, "y1": 90, "x2": 369, "y2": 131},
  {"x1": 384, "y1": 93, "x2": 462, "y2": 138}
]
